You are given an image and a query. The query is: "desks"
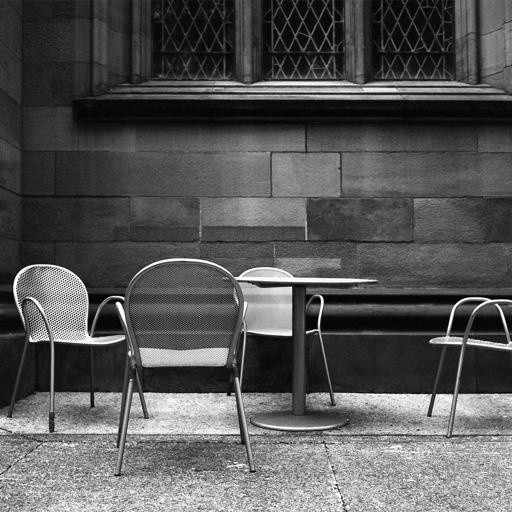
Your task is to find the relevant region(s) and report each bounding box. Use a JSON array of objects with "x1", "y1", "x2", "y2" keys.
[{"x1": 225, "y1": 278, "x2": 377, "y2": 432}]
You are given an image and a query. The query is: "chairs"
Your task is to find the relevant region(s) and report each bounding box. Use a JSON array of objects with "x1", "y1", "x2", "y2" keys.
[
  {"x1": 114, "y1": 259, "x2": 257, "y2": 475},
  {"x1": 428, "y1": 295, "x2": 512, "y2": 438},
  {"x1": 7, "y1": 264, "x2": 126, "y2": 431},
  {"x1": 232, "y1": 267, "x2": 338, "y2": 407}
]
